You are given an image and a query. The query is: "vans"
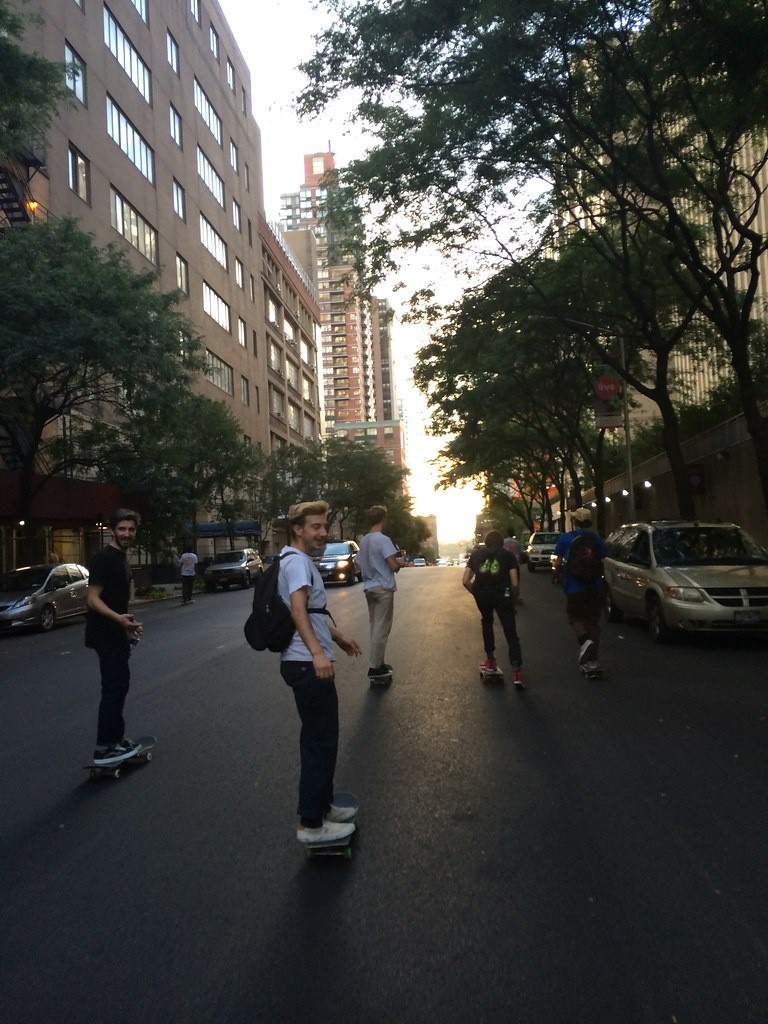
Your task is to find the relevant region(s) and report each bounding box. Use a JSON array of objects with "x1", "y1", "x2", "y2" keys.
[{"x1": 414, "y1": 558, "x2": 426, "y2": 566}]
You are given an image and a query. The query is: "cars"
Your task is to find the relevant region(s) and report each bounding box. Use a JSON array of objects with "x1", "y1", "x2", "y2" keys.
[
  {"x1": 548, "y1": 551, "x2": 566, "y2": 583},
  {"x1": 0, "y1": 563, "x2": 89, "y2": 632},
  {"x1": 262, "y1": 554, "x2": 282, "y2": 571}
]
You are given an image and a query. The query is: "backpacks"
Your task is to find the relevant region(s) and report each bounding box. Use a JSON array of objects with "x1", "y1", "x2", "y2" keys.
[
  {"x1": 244, "y1": 553, "x2": 332, "y2": 654},
  {"x1": 567, "y1": 533, "x2": 600, "y2": 574},
  {"x1": 476, "y1": 551, "x2": 507, "y2": 585}
]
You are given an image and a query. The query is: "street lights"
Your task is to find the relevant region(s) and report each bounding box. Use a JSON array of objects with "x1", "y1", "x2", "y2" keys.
[{"x1": 527, "y1": 313, "x2": 637, "y2": 520}]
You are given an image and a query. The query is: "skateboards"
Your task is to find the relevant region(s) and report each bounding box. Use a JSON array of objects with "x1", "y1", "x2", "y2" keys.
[
  {"x1": 580, "y1": 660, "x2": 605, "y2": 680},
  {"x1": 479, "y1": 664, "x2": 504, "y2": 682},
  {"x1": 369, "y1": 663, "x2": 393, "y2": 687},
  {"x1": 82, "y1": 737, "x2": 158, "y2": 778},
  {"x1": 304, "y1": 790, "x2": 359, "y2": 860}
]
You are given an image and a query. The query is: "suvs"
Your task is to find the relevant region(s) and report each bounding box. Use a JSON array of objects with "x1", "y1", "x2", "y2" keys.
[
  {"x1": 602, "y1": 516, "x2": 768, "y2": 645},
  {"x1": 310, "y1": 540, "x2": 363, "y2": 586},
  {"x1": 525, "y1": 532, "x2": 564, "y2": 572},
  {"x1": 204, "y1": 547, "x2": 263, "y2": 594}
]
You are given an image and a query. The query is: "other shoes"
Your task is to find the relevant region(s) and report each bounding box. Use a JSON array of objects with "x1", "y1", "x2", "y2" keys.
[{"x1": 578, "y1": 639, "x2": 599, "y2": 669}]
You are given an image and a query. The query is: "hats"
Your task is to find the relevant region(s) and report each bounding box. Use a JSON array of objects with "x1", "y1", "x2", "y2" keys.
[{"x1": 568, "y1": 507, "x2": 594, "y2": 525}]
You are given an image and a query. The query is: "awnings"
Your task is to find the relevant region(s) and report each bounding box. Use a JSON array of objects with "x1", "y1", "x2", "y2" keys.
[{"x1": 181, "y1": 520, "x2": 261, "y2": 548}]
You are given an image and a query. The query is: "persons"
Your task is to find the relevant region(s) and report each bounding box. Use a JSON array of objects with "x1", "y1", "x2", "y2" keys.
[
  {"x1": 357, "y1": 505, "x2": 399, "y2": 678},
  {"x1": 179, "y1": 546, "x2": 198, "y2": 602},
  {"x1": 85, "y1": 509, "x2": 145, "y2": 765},
  {"x1": 463, "y1": 532, "x2": 526, "y2": 688},
  {"x1": 503, "y1": 528, "x2": 522, "y2": 598},
  {"x1": 555, "y1": 508, "x2": 605, "y2": 669},
  {"x1": 276, "y1": 499, "x2": 356, "y2": 843}
]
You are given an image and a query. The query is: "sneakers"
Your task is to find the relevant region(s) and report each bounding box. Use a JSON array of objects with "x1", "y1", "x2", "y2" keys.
[
  {"x1": 296, "y1": 819, "x2": 356, "y2": 844},
  {"x1": 479, "y1": 659, "x2": 497, "y2": 673},
  {"x1": 93, "y1": 735, "x2": 143, "y2": 765},
  {"x1": 513, "y1": 671, "x2": 526, "y2": 690},
  {"x1": 325, "y1": 803, "x2": 357, "y2": 823},
  {"x1": 367, "y1": 664, "x2": 394, "y2": 679}
]
